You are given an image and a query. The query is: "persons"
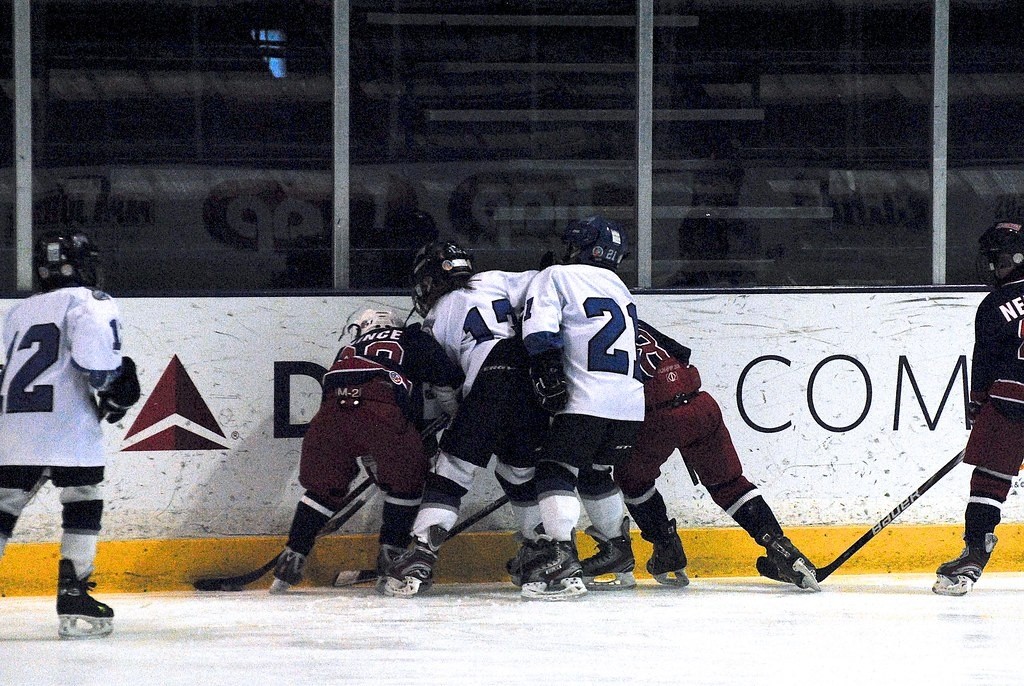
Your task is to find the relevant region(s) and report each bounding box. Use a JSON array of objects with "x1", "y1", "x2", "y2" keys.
[
  {"x1": 375, "y1": 239, "x2": 550, "y2": 598},
  {"x1": 932, "y1": 219, "x2": 1024, "y2": 596},
  {"x1": 613, "y1": 318, "x2": 822, "y2": 592},
  {"x1": 285, "y1": 208, "x2": 440, "y2": 288},
  {"x1": 521, "y1": 216, "x2": 646, "y2": 601},
  {"x1": 269, "y1": 307, "x2": 467, "y2": 597},
  {"x1": 0, "y1": 228, "x2": 141, "y2": 639},
  {"x1": 680, "y1": 152, "x2": 786, "y2": 288}
]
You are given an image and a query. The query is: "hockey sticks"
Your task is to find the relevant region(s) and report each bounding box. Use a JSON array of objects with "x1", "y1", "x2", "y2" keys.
[
  {"x1": 326, "y1": 489, "x2": 515, "y2": 591},
  {"x1": 194, "y1": 415, "x2": 450, "y2": 598},
  {"x1": 753, "y1": 440, "x2": 971, "y2": 589}
]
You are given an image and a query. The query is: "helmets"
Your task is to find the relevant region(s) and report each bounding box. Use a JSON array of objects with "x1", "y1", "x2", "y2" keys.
[
  {"x1": 975, "y1": 222, "x2": 1024, "y2": 290},
  {"x1": 348, "y1": 308, "x2": 404, "y2": 341},
  {"x1": 409, "y1": 240, "x2": 473, "y2": 319},
  {"x1": 561, "y1": 215, "x2": 629, "y2": 271},
  {"x1": 35, "y1": 227, "x2": 104, "y2": 291}
]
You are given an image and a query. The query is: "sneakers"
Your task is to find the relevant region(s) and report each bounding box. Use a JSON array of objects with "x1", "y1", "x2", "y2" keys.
[
  {"x1": 754, "y1": 523, "x2": 823, "y2": 594},
  {"x1": 581, "y1": 516, "x2": 637, "y2": 591},
  {"x1": 510, "y1": 528, "x2": 587, "y2": 601},
  {"x1": 55, "y1": 559, "x2": 115, "y2": 639},
  {"x1": 267, "y1": 551, "x2": 305, "y2": 596},
  {"x1": 931, "y1": 532, "x2": 999, "y2": 596},
  {"x1": 640, "y1": 518, "x2": 690, "y2": 587},
  {"x1": 503, "y1": 522, "x2": 550, "y2": 587},
  {"x1": 375, "y1": 535, "x2": 438, "y2": 597}
]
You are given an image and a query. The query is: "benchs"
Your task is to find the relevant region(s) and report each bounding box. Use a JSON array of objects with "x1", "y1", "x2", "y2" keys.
[{"x1": 362, "y1": 0, "x2": 839, "y2": 274}]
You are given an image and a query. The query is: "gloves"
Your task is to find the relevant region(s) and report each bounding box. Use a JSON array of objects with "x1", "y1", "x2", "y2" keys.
[{"x1": 95, "y1": 356, "x2": 141, "y2": 424}]
